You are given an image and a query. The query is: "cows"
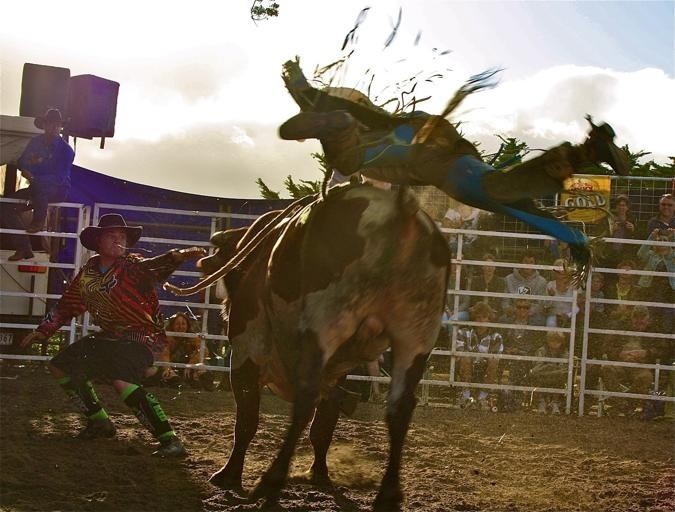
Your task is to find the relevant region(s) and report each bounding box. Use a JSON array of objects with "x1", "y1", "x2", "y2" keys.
[{"x1": 196, "y1": 63, "x2": 503, "y2": 507}]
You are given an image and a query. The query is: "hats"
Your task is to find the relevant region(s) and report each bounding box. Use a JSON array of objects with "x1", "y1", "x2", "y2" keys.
[
  {"x1": 80, "y1": 214, "x2": 142, "y2": 251},
  {"x1": 35, "y1": 109, "x2": 71, "y2": 129}
]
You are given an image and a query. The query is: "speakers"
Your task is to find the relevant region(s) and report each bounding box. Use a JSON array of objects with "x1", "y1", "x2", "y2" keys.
[
  {"x1": 64, "y1": 74, "x2": 120, "y2": 138},
  {"x1": 19, "y1": 63, "x2": 70, "y2": 119}
]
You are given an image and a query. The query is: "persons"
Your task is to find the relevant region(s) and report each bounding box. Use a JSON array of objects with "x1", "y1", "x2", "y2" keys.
[
  {"x1": 143, "y1": 194, "x2": 675, "y2": 420},
  {"x1": 274, "y1": 52, "x2": 634, "y2": 267},
  {"x1": 19, "y1": 211, "x2": 207, "y2": 458},
  {"x1": 0, "y1": 107, "x2": 76, "y2": 263}
]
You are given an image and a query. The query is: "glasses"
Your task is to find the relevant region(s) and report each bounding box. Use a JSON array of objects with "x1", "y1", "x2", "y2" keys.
[
  {"x1": 660, "y1": 201, "x2": 673, "y2": 207},
  {"x1": 516, "y1": 303, "x2": 529, "y2": 311}
]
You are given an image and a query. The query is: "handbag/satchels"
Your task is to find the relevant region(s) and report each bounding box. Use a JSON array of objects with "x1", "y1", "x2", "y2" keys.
[
  {"x1": 644, "y1": 389, "x2": 666, "y2": 418},
  {"x1": 200, "y1": 356, "x2": 224, "y2": 390}
]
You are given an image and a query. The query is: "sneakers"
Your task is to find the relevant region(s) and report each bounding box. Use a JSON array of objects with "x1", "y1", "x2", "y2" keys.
[
  {"x1": 152, "y1": 435, "x2": 186, "y2": 459},
  {"x1": 73, "y1": 418, "x2": 115, "y2": 440},
  {"x1": 26, "y1": 217, "x2": 47, "y2": 232},
  {"x1": 9, "y1": 246, "x2": 34, "y2": 260},
  {"x1": 279, "y1": 108, "x2": 357, "y2": 141},
  {"x1": 587, "y1": 122, "x2": 630, "y2": 177}
]
